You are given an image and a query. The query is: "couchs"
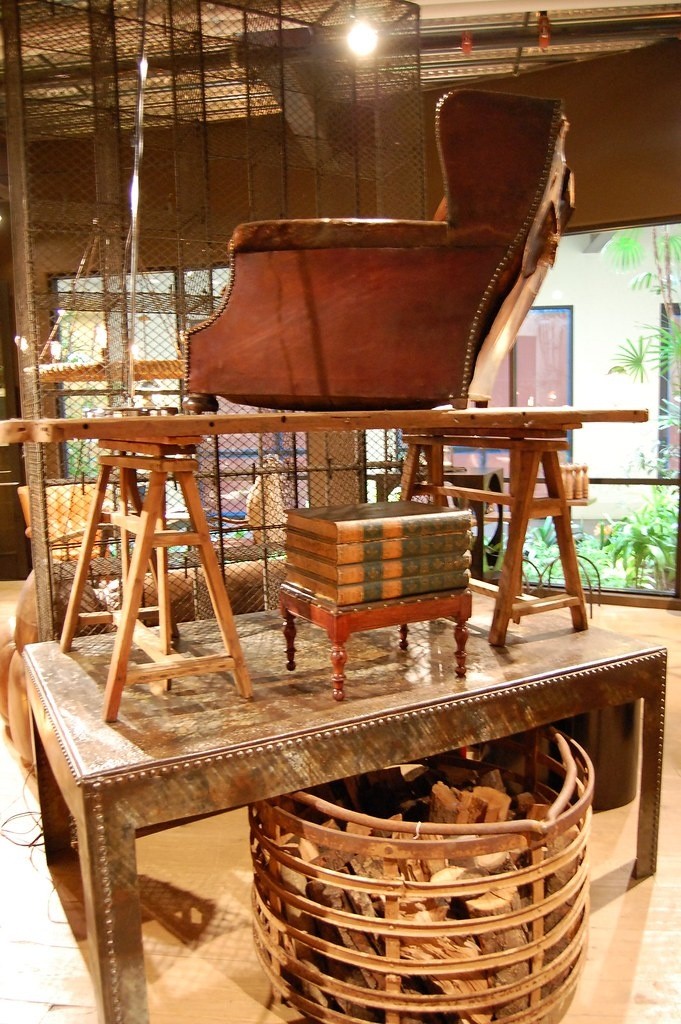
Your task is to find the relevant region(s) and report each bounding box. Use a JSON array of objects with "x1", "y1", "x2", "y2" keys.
[
  {"x1": 0, "y1": 556, "x2": 290, "y2": 765},
  {"x1": 17, "y1": 484, "x2": 113, "y2": 564}
]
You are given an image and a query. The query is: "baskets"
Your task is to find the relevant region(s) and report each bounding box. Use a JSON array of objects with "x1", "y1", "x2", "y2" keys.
[{"x1": 251, "y1": 724, "x2": 595, "y2": 1024}]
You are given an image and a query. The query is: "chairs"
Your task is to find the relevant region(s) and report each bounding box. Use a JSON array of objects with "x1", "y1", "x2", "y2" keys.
[{"x1": 182, "y1": 89, "x2": 577, "y2": 416}]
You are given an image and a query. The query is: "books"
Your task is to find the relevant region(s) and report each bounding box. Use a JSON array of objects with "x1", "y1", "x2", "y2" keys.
[{"x1": 282, "y1": 502, "x2": 472, "y2": 607}]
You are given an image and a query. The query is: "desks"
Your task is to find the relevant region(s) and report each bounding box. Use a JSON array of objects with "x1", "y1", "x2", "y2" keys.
[
  {"x1": 22, "y1": 592, "x2": 668, "y2": 1024},
  {"x1": 278, "y1": 582, "x2": 473, "y2": 699},
  {"x1": 0, "y1": 410, "x2": 651, "y2": 722}
]
